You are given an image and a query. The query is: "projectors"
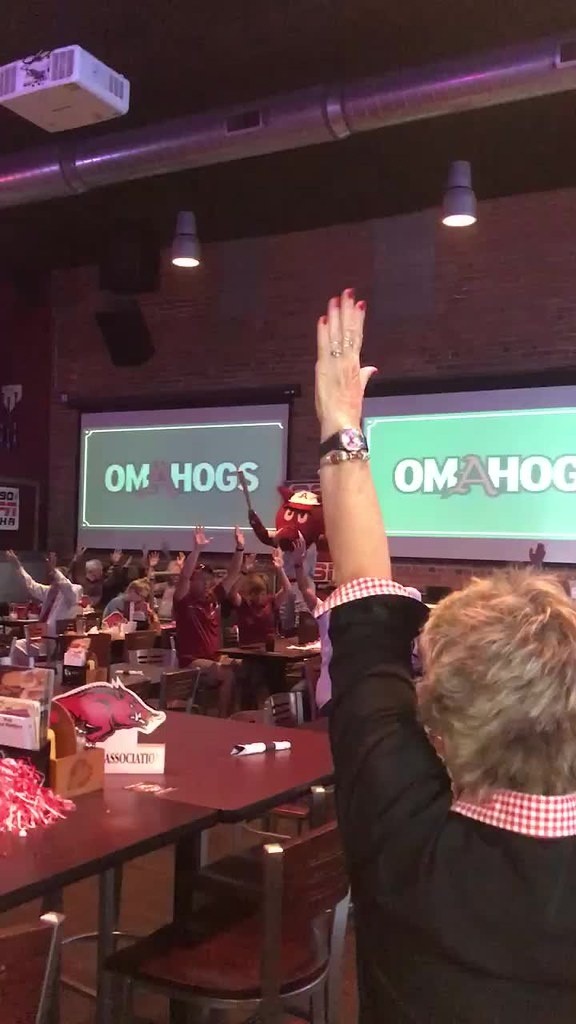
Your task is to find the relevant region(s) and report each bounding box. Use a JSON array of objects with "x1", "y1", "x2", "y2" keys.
[{"x1": 0, "y1": 44, "x2": 130, "y2": 134}]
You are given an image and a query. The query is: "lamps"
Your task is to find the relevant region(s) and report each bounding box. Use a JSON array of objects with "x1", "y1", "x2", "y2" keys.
[
  {"x1": 170, "y1": 210, "x2": 202, "y2": 268},
  {"x1": 441, "y1": 160, "x2": 478, "y2": 228}
]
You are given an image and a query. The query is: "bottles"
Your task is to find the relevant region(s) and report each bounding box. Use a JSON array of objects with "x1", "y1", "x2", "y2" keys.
[
  {"x1": 75, "y1": 614, "x2": 83, "y2": 634},
  {"x1": 266, "y1": 629, "x2": 274, "y2": 652}
]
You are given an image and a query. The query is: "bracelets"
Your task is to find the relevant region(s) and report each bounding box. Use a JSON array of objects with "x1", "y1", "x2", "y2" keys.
[
  {"x1": 235, "y1": 547, "x2": 245, "y2": 551},
  {"x1": 316, "y1": 450, "x2": 369, "y2": 477},
  {"x1": 293, "y1": 562, "x2": 304, "y2": 568}
]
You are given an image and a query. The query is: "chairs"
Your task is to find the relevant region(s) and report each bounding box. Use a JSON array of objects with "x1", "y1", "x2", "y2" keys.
[{"x1": 0, "y1": 595, "x2": 351, "y2": 1024}]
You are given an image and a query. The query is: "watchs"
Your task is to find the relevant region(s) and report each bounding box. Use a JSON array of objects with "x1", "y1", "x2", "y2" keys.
[{"x1": 319, "y1": 427, "x2": 369, "y2": 459}]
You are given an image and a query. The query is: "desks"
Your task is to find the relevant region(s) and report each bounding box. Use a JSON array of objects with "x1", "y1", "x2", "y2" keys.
[
  {"x1": 105, "y1": 710, "x2": 334, "y2": 1024},
  {"x1": 11, "y1": 630, "x2": 162, "y2": 662},
  {"x1": 52, "y1": 656, "x2": 217, "y2": 699},
  {"x1": 1, "y1": 607, "x2": 96, "y2": 638},
  {"x1": 0, "y1": 711, "x2": 221, "y2": 1024},
  {"x1": 215, "y1": 634, "x2": 321, "y2": 693},
  {"x1": 159, "y1": 619, "x2": 176, "y2": 648}
]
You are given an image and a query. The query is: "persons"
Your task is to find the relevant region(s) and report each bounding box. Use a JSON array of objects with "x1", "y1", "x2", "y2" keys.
[
  {"x1": 3, "y1": 525, "x2": 293, "y2": 716},
  {"x1": 313, "y1": 287, "x2": 575, "y2": 1023},
  {"x1": 292, "y1": 530, "x2": 333, "y2": 712}
]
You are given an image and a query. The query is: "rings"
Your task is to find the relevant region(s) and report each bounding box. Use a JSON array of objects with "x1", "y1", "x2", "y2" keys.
[
  {"x1": 344, "y1": 337, "x2": 354, "y2": 348},
  {"x1": 331, "y1": 341, "x2": 342, "y2": 356}
]
